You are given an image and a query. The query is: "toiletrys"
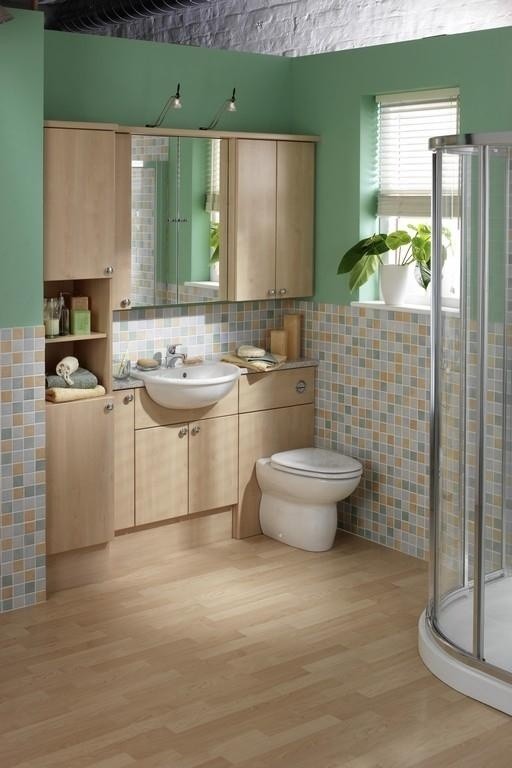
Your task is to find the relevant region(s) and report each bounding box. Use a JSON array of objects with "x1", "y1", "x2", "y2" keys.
[{"x1": 44, "y1": 292, "x2": 70, "y2": 339}]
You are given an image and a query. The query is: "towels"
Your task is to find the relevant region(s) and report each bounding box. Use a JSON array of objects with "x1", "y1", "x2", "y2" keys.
[{"x1": 43, "y1": 355, "x2": 105, "y2": 403}]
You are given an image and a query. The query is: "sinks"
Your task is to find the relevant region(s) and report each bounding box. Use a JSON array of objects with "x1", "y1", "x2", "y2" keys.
[{"x1": 144, "y1": 360, "x2": 240, "y2": 409}]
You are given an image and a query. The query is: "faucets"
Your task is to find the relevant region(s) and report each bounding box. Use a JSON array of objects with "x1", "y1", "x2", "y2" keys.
[{"x1": 163, "y1": 343, "x2": 187, "y2": 368}]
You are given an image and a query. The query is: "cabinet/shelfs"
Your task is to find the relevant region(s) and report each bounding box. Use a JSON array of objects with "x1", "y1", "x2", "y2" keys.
[
  {"x1": 111, "y1": 133, "x2": 131, "y2": 312},
  {"x1": 110, "y1": 388, "x2": 136, "y2": 530},
  {"x1": 232, "y1": 365, "x2": 315, "y2": 542},
  {"x1": 45, "y1": 398, "x2": 117, "y2": 555},
  {"x1": 40, "y1": 127, "x2": 117, "y2": 279},
  {"x1": 230, "y1": 138, "x2": 313, "y2": 300},
  {"x1": 43, "y1": 275, "x2": 112, "y2": 405},
  {"x1": 135, "y1": 414, "x2": 238, "y2": 525}
]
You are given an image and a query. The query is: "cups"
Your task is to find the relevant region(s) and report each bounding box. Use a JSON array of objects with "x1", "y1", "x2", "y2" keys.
[{"x1": 112, "y1": 359, "x2": 130, "y2": 379}]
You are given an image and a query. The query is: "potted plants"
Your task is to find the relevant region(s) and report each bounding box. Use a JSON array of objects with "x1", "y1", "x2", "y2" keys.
[{"x1": 335, "y1": 222, "x2": 450, "y2": 306}]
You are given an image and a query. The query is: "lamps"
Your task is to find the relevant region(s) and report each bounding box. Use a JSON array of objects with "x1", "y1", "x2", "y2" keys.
[{"x1": 146, "y1": 82, "x2": 238, "y2": 130}]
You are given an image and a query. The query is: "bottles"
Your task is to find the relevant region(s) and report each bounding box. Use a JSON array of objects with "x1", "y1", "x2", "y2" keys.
[{"x1": 43, "y1": 293, "x2": 71, "y2": 339}]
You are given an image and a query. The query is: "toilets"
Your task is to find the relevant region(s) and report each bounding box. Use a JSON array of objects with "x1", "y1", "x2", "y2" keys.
[{"x1": 255, "y1": 447, "x2": 363, "y2": 552}]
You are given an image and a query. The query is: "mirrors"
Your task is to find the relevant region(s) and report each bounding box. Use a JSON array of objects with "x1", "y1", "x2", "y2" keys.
[{"x1": 130, "y1": 134, "x2": 230, "y2": 308}]
